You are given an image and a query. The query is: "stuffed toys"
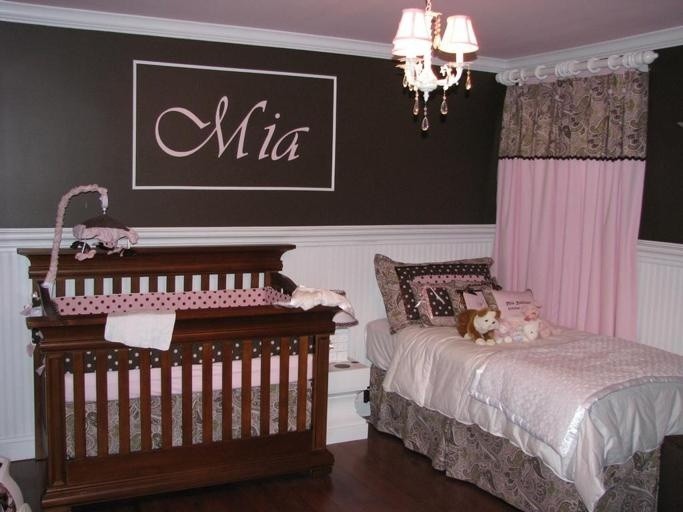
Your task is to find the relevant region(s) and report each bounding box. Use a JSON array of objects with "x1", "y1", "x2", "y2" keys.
[{"x1": 455, "y1": 302, "x2": 562, "y2": 346}]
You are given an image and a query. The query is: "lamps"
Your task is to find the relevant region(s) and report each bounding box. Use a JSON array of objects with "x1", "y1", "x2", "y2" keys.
[
  {"x1": 27, "y1": 184, "x2": 139, "y2": 317},
  {"x1": 392, "y1": 0, "x2": 479, "y2": 130}
]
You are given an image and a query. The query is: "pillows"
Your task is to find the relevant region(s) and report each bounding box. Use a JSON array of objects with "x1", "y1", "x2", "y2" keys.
[{"x1": 373, "y1": 253, "x2": 538, "y2": 335}]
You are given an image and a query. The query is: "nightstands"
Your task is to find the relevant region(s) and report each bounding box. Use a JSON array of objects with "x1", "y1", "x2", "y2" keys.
[{"x1": 326, "y1": 357, "x2": 370, "y2": 446}]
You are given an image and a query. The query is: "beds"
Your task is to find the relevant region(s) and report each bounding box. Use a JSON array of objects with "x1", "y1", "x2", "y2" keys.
[
  {"x1": 18, "y1": 243, "x2": 342, "y2": 512},
  {"x1": 367, "y1": 316, "x2": 683, "y2": 512}
]
